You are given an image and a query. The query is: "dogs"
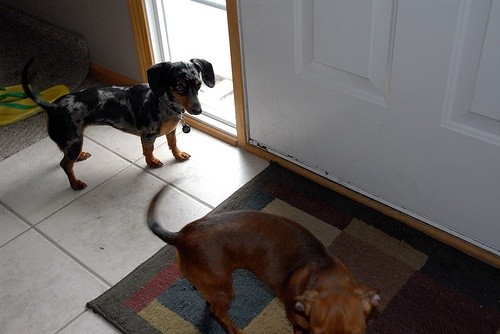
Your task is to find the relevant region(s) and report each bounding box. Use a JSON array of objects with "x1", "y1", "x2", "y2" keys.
[
  {"x1": 21, "y1": 54, "x2": 215, "y2": 191},
  {"x1": 146, "y1": 177, "x2": 382, "y2": 334}
]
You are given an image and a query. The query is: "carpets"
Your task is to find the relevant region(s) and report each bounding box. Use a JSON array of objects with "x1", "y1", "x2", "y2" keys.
[
  {"x1": 83, "y1": 163, "x2": 500, "y2": 334},
  {"x1": 0, "y1": 21, "x2": 90, "y2": 165}
]
